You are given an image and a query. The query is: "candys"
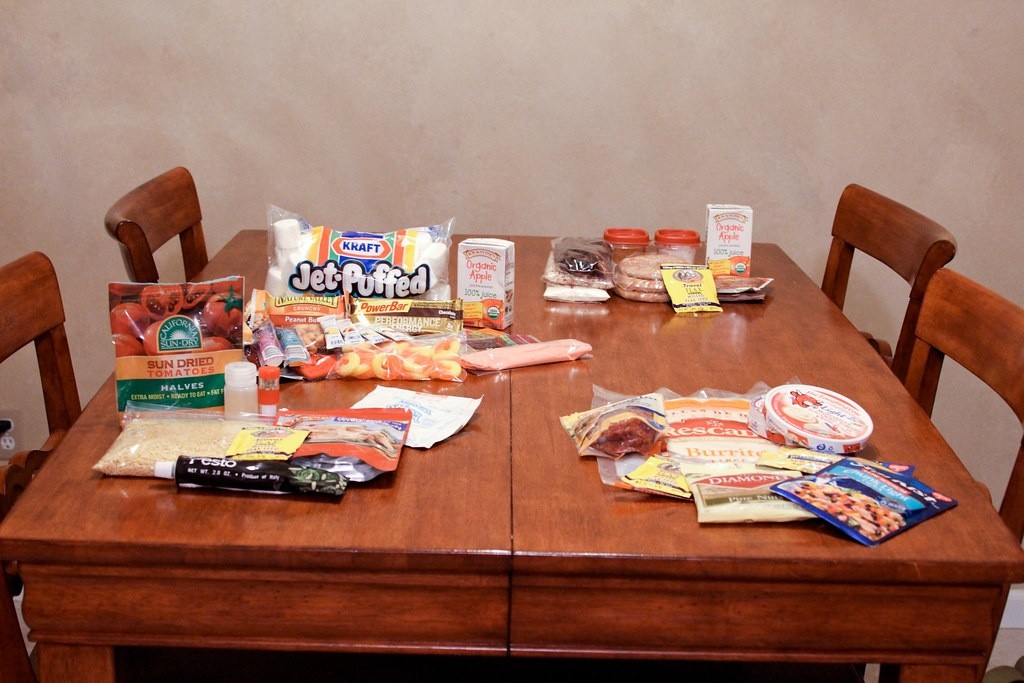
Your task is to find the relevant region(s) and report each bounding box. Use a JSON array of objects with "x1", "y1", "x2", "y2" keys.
[{"x1": 334, "y1": 337, "x2": 463, "y2": 382}]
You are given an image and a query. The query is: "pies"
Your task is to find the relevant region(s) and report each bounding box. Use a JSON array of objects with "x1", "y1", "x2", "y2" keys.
[{"x1": 613, "y1": 253, "x2": 689, "y2": 303}]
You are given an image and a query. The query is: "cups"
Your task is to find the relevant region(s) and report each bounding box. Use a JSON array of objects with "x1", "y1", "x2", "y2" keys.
[
  {"x1": 602, "y1": 228, "x2": 650, "y2": 264},
  {"x1": 653, "y1": 228, "x2": 704, "y2": 265}
]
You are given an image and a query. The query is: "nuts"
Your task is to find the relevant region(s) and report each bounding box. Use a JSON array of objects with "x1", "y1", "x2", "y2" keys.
[{"x1": 294, "y1": 323, "x2": 325, "y2": 351}]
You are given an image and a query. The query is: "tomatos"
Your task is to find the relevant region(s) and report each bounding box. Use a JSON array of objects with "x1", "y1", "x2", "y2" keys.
[{"x1": 108, "y1": 277, "x2": 244, "y2": 357}]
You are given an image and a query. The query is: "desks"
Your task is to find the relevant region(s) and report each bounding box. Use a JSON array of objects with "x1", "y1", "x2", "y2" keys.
[
  {"x1": 1, "y1": 230, "x2": 512, "y2": 682},
  {"x1": 513, "y1": 235, "x2": 1020, "y2": 683}
]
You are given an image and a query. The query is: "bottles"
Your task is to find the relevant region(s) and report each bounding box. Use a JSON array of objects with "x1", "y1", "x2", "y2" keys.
[
  {"x1": 223, "y1": 362, "x2": 258, "y2": 415},
  {"x1": 257, "y1": 366, "x2": 280, "y2": 416}
]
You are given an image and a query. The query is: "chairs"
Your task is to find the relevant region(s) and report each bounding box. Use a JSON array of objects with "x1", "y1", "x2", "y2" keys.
[
  {"x1": 819, "y1": 184, "x2": 959, "y2": 377},
  {"x1": 106, "y1": 167, "x2": 212, "y2": 324},
  {"x1": 2, "y1": 250, "x2": 82, "y2": 679},
  {"x1": 864, "y1": 268, "x2": 1022, "y2": 680}
]
